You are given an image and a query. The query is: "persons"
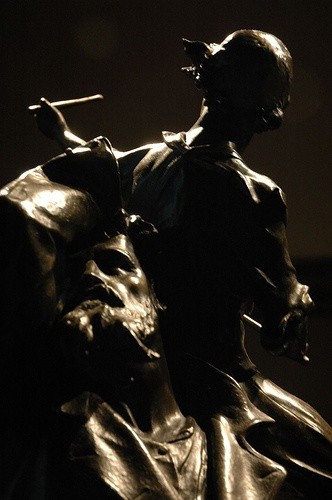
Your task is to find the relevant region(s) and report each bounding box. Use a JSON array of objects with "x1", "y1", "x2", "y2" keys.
[
  {"x1": 1, "y1": 135, "x2": 332, "y2": 498},
  {"x1": 27, "y1": 30, "x2": 312, "y2": 383}
]
